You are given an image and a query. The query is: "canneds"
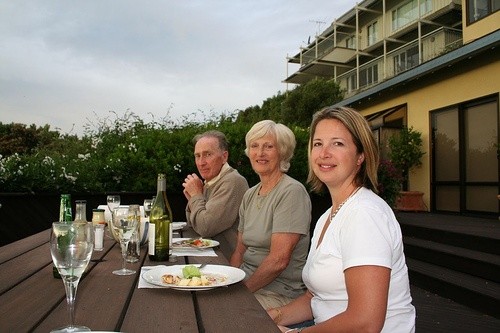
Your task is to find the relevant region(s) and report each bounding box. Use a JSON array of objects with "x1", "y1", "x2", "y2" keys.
[{"x1": 91, "y1": 209, "x2": 105, "y2": 230}]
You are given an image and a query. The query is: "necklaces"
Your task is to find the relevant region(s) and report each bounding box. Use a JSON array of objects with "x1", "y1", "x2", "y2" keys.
[{"x1": 329, "y1": 183, "x2": 360, "y2": 222}]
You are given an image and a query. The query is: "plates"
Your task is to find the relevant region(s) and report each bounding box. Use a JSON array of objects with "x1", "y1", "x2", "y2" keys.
[
  {"x1": 172, "y1": 221, "x2": 187, "y2": 230},
  {"x1": 141, "y1": 263, "x2": 246, "y2": 291},
  {"x1": 172, "y1": 237, "x2": 220, "y2": 251}
]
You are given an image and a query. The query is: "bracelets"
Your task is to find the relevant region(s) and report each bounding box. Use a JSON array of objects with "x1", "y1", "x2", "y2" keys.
[
  {"x1": 266, "y1": 307, "x2": 281, "y2": 327},
  {"x1": 297, "y1": 326, "x2": 303, "y2": 333}
]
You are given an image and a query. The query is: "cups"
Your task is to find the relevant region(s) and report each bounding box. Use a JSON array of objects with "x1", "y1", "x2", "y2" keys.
[
  {"x1": 144, "y1": 200, "x2": 154, "y2": 217},
  {"x1": 107, "y1": 196, "x2": 120, "y2": 213},
  {"x1": 94, "y1": 224, "x2": 105, "y2": 251}
]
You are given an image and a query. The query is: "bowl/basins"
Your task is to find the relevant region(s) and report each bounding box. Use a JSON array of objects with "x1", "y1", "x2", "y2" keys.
[{"x1": 111, "y1": 220, "x2": 149, "y2": 245}]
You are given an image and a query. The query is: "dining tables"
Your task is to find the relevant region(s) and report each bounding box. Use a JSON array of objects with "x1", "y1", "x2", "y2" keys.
[{"x1": 0, "y1": 222, "x2": 284, "y2": 333}]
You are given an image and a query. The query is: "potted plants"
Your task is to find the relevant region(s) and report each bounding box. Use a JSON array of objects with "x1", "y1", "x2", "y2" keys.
[{"x1": 387, "y1": 125, "x2": 427, "y2": 212}]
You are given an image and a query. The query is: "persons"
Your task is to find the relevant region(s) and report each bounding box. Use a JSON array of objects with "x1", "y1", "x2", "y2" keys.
[
  {"x1": 179, "y1": 129, "x2": 250, "y2": 263},
  {"x1": 247, "y1": 106, "x2": 418, "y2": 333},
  {"x1": 227, "y1": 121, "x2": 312, "y2": 317}
]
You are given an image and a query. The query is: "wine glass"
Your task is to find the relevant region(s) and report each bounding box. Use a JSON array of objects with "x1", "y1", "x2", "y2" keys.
[
  {"x1": 111, "y1": 206, "x2": 136, "y2": 276},
  {"x1": 49, "y1": 222, "x2": 95, "y2": 333}
]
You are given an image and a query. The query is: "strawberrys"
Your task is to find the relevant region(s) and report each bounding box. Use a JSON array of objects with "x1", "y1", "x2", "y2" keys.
[{"x1": 194, "y1": 240, "x2": 203, "y2": 247}]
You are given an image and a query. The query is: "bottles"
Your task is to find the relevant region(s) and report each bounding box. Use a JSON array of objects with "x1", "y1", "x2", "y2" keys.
[
  {"x1": 53, "y1": 194, "x2": 73, "y2": 278},
  {"x1": 148, "y1": 174, "x2": 173, "y2": 262},
  {"x1": 92, "y1": 209, "x2": 105, "y2": 224},
  {"x1": 126, "y1": 205, "x2": 140, "y2": 263},
  {"x1": 72, "y1": 199, "x2": 88, "y2": 242}
]
